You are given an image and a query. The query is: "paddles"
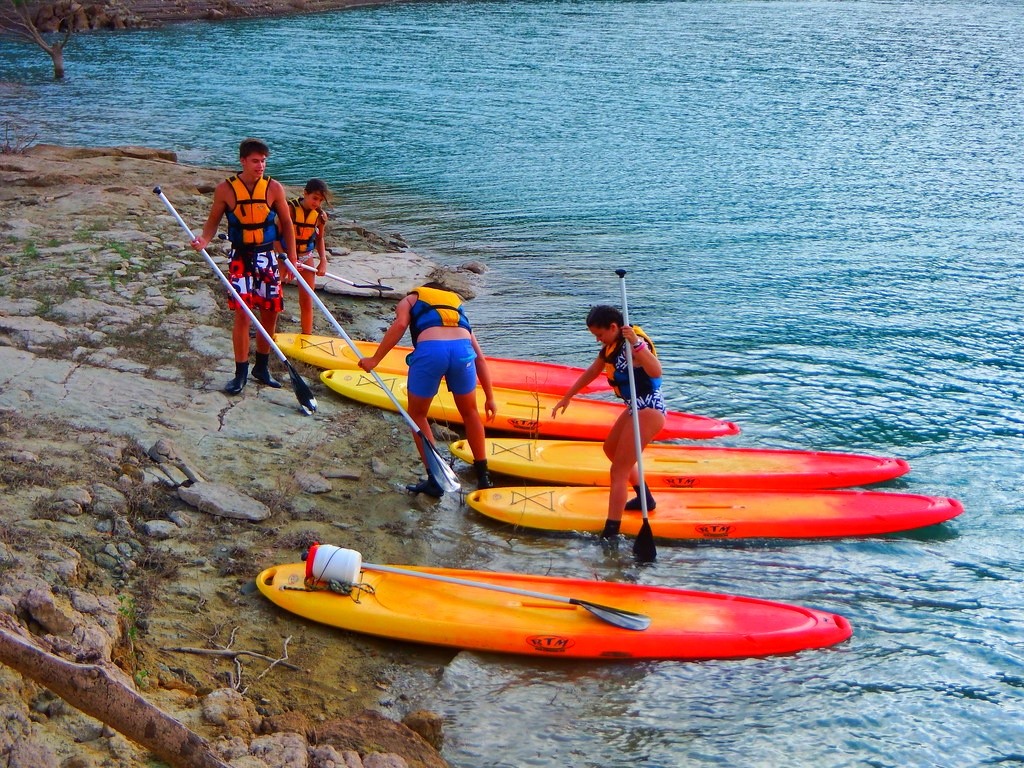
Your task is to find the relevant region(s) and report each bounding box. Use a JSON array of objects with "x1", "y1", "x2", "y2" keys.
[
  {"x1": 616, "y1": 267, "x2": 660, "y2": 566},
  {"x1": 300, "y1": 551, "x2": 650, "y2": 632},
  {"x1": 297, "y1": 259, "x2": 394, "y2": 291},
  {"x1": 280, "y1": 252, "x2": 461, "y2": 494},
  {"x1": 152, "y1": 185, "x2": 318, "y2": 419}
]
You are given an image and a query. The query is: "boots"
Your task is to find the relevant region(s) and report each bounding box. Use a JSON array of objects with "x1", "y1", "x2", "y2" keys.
[
  {"x1": 225, "y1": 361, "x2": 249, "y2": 394},
  {"x1": 406, "y1": 467, "x2": 444, "y2": 498},
  {"x1": 250, "y1": 351, "x2": 282, "y2": 388},
  {"x1": 599, "y1": 519, "x2": 621, "y2": 549},
  {"x1": 473, "y1": 459, "x2": 494, "y2": 488},
  {"x1": 625, "y1": 481, "x2": 656, "y2": 510}
]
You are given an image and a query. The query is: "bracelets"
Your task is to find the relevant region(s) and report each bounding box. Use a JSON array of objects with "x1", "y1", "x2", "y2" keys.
[{"x1": 631, "y1": 337, "x2": 647, "y2": 352}]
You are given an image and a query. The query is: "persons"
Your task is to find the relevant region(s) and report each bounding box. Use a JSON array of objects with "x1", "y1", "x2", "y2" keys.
[
  {"x1": 277, "y1": 178, "x2": 327, "y2": 335},
  {"x1": 551, "y1": 303, "x2": 665, "y2": 545},
  {"x1": 358, "y1": 282, "x2": 496, "y2": 498},
  {"x1": 188, "y1": 137, "x2": 297, "y2": 395}
]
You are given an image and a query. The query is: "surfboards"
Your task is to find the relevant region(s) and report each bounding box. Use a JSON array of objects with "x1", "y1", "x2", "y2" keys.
[
  {"x1": 253, "y1": 555, "x2": 855, "y2": 659},
  {"x1": 261, "y1": 320, "x2": 619, "y2": 390},
  {"x1": 311, "y1": 366, "x2": 740, "y2": 442},
  {"x1": 446, "y1": 433, "x2": 964, "y2": 543}
]
